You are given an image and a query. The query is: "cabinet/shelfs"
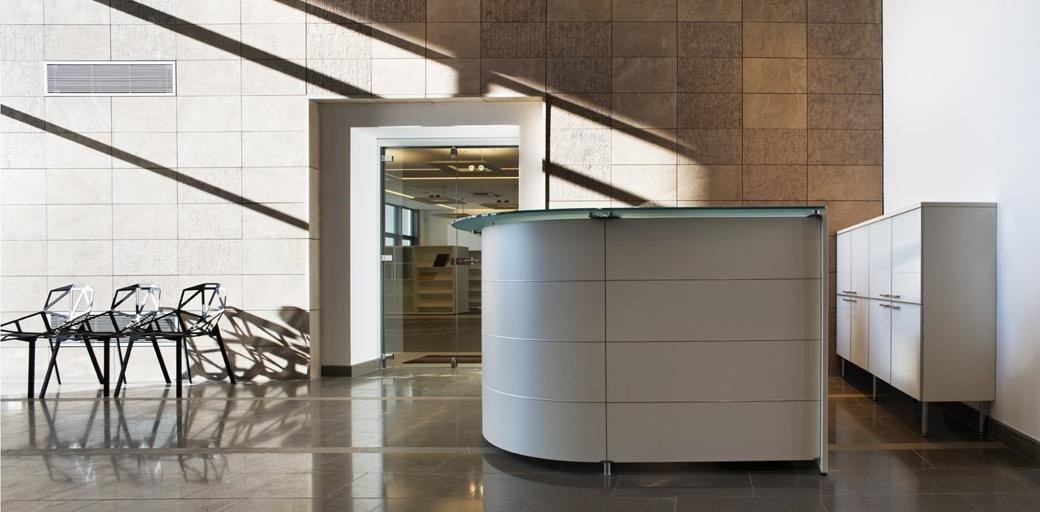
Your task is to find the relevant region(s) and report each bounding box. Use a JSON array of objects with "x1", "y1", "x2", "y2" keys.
[
  {"x1": 868, "y1": 202, "x2": 1003, "y2": 435},
  {"x1": 382, "y1": 245, "x2": 483, "y2": 317},
  {"x1": 835, "y1": 226, "x2": 876, "y2": 402}
]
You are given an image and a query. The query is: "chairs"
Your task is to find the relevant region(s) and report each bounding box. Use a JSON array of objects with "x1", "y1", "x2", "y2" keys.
[
  {"x1": 114, "y1": 281, "x2": 234, "y2": 397},
  {"x1": 35, "y1": 282, "x2": 171, "y2": 400},
  {"x1": 0, "y1": 279, "x2": 104, "y2": 396}
]
